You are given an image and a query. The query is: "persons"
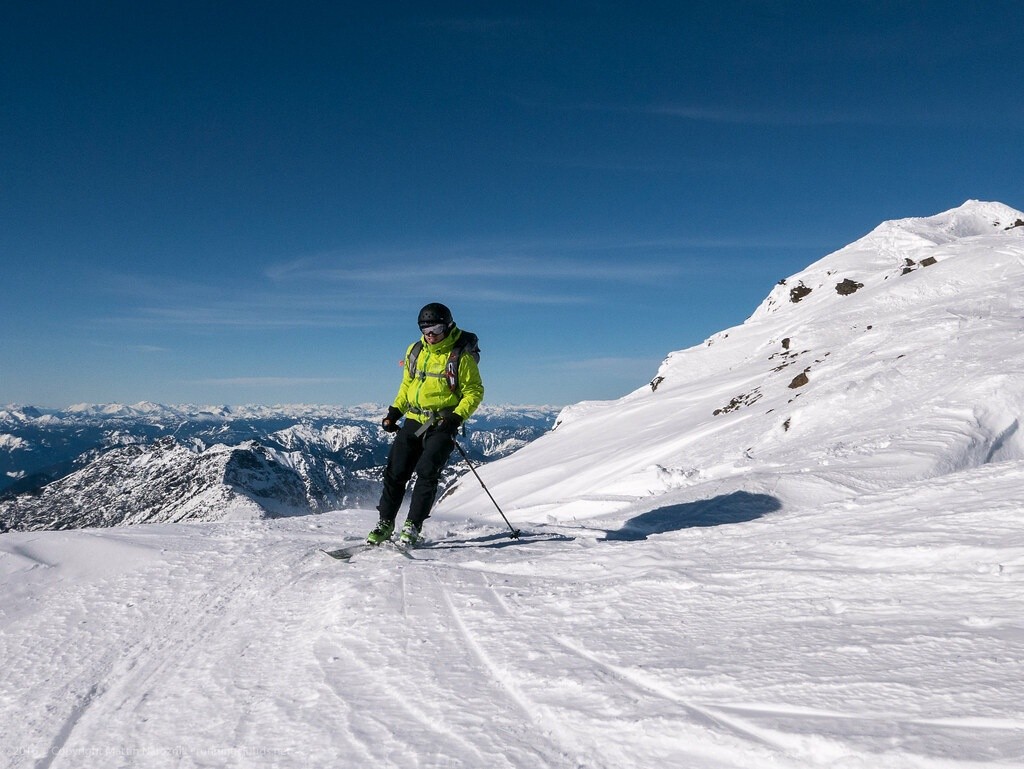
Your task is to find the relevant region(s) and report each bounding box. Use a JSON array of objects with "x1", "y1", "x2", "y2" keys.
[{"x1": 366, "y1": 301, "x2": 484, "y2": 546}]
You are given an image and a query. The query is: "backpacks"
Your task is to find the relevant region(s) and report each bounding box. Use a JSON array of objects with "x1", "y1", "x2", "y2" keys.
[{"x1": 407, "y1": 327, "x2": 481, "y2": 436}]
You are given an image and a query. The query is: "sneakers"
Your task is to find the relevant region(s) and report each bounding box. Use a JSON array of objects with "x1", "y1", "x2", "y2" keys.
[
  {"x1": 367, "y1": 518, "x2": 394, "y2": 544},
  {"x1": 400, "y1": 518, "x2": 419, "y2": 544}
]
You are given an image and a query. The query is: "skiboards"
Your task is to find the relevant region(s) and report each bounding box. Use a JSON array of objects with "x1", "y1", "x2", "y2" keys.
[
  {"x1": 319, "y1": 543, "x2": 373, "y2": 560},
  {"x1": 377, "y1": 539, "x2": 416, "y2": 560}
]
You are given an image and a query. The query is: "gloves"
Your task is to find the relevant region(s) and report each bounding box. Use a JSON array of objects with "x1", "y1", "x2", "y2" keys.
[
  {"x1": 432, "y1": 412, "x2": 464, "y2": 436},
  {"x1": 382, "y1": 405, "x2": 404, "y2": 432}
]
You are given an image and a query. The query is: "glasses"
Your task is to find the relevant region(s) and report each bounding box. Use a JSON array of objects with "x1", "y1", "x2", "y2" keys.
[{"x1": 421, "y1": 323, "x2": 444, "y2": 335}]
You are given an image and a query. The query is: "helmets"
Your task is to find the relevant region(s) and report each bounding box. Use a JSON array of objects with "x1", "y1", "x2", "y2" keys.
[{"x1": 418, "y1": 302, "x2": 456, "y2": 336}]
